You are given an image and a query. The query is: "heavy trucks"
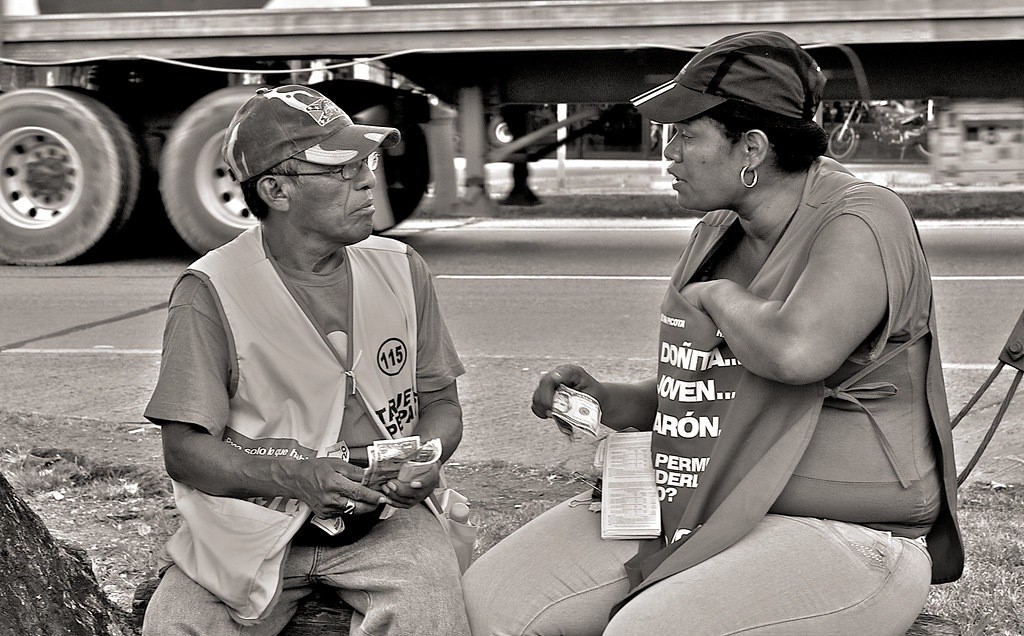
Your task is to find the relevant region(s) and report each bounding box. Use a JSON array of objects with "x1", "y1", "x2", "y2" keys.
[{"x1": 0, "y1": 0, "x2": 1024, "y2": 267}]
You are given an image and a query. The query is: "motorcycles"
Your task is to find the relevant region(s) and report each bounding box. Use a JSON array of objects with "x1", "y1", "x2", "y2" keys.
[{"x1": 827, "y1": 98, "x2": 930, "y2": 160}]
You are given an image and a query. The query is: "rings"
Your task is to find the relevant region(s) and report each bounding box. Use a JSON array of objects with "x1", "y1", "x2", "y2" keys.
[
  {"x1": 343, "y1": 499, "x2": 356, "y2": 515},
  {"x1": 550, "y1": 371, "x2": 563, "y2": 385}
]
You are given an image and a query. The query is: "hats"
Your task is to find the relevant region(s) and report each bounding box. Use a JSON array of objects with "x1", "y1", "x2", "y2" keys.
[
  {"x1": 221, "y1": 84, "x2": 402, "y2": 183},
  {"x1": 629, "y1": 31, "x2": 828, "y2": 124}
]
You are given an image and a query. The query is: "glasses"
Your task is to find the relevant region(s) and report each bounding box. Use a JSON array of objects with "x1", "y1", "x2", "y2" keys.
[{"x1": 249, "y1": 151, "x2": 381, "y2": 181}]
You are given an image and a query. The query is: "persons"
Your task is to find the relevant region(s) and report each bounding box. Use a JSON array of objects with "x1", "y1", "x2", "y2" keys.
[
  {"x1": 141, "y1": 83, "x2": 472, "y2": 636},
  {"x1": 462, "y1": 30, "x2": 965, "y2": 635}
]
